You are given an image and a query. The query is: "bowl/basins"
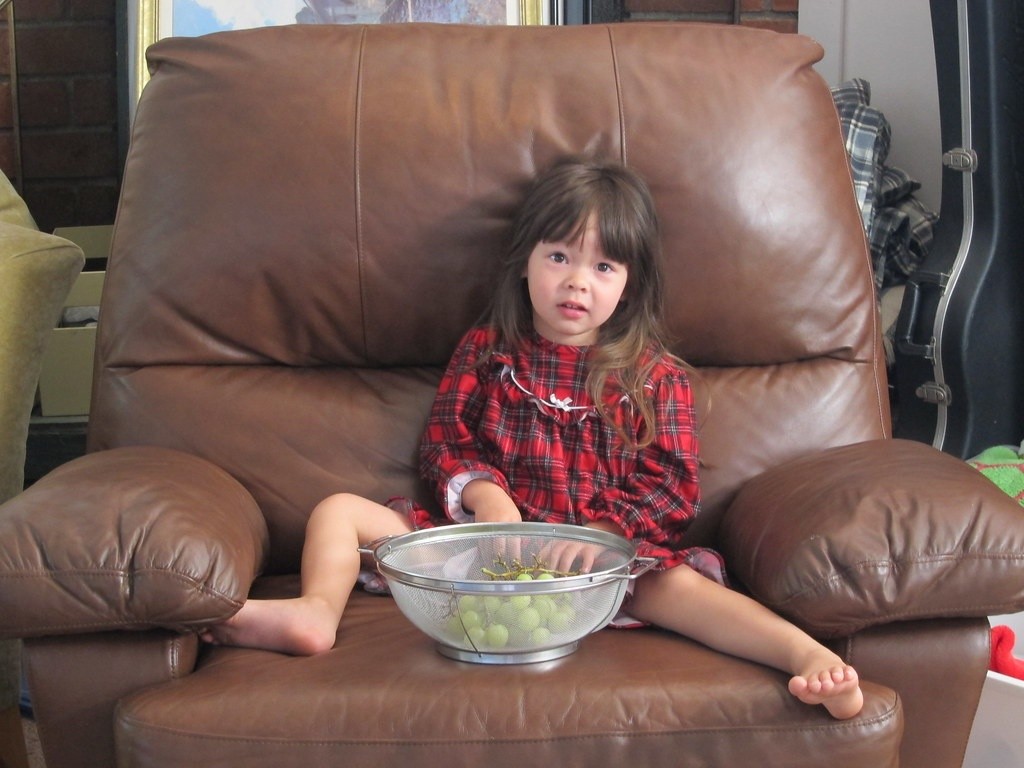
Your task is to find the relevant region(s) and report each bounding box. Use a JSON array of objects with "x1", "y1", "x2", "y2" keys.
[{"x1": 356, "y1": 521, "x2": 659, "y2": 664}]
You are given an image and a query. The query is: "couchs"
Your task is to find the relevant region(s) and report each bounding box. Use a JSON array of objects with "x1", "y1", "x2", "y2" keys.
[{"x1": 0, "y1": 22, "x2": 1024, "y2": 768}]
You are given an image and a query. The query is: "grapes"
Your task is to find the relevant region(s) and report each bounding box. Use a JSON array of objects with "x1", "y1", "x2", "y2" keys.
[{"x1": 449, "y1": 571, "x2": 576, "y2": 649}]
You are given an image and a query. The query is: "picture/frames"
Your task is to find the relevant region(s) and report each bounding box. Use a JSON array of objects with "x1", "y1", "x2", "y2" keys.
[{"x1": 136, "y1": 0, "x2": 544, "y2": 107}]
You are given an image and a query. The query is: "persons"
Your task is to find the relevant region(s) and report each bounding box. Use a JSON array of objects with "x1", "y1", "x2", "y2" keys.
[{"x1": 200, "y1": 154, "x2": 863, "y2": 719}]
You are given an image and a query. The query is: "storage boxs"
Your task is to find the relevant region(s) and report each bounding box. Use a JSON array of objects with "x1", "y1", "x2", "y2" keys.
[{"x1": 38, "y1": 225, "x2": 114, "y2": 416}]
